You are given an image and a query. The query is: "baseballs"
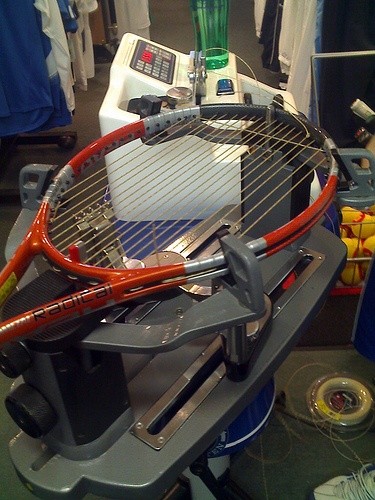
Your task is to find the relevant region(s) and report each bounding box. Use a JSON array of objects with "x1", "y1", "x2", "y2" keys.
[{"x1": 338, "y1": 205, "x2": 375, "y2": 285}]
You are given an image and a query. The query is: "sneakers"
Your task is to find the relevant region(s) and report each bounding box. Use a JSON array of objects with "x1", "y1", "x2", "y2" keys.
[{"x1": 311, "y1": 463, "x2": 375, "y2": 500}]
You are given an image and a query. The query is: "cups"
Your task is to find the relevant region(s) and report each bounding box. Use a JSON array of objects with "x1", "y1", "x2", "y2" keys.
[{"x1": 190, "y1": 1, "x2": 229, "y2": 69}]
